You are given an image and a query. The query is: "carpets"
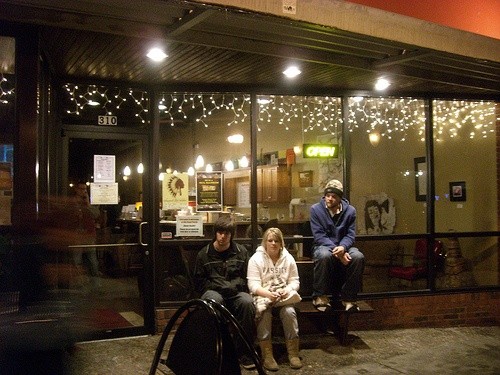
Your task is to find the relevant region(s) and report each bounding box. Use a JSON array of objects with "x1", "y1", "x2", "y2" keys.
[{"x1": 74, "y1": 309, "x2": 134, "y2": 330}]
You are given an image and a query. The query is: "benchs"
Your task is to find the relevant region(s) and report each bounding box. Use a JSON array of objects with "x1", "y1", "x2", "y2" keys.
[{"x1": 229, "y1": 261, "x2": 374, "y2": 349}]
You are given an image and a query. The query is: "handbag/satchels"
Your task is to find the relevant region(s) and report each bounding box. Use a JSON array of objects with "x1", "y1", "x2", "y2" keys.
[{"x1": 273, "y1": 290, "x2": 302, "y2": 306}]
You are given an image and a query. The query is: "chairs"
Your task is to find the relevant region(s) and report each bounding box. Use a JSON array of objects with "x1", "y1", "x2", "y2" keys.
[
  {"x1": 149, "y1": 298, "x2": 267, "y2": 375},
  {"x1": 386, "y1": 239, "x2": 443, "y2": 291}
]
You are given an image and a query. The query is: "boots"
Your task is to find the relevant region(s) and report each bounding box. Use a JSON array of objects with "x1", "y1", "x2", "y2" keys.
[
  {"x1": 287, "y1": 337, "x2": 302, "y2": 368},
  {"x1": 260, "y1": 339, "x2": 279, "y2": 371}
]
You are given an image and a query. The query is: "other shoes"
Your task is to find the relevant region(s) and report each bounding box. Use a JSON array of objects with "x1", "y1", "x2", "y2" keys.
[
  {"x1": 95, "y1": 282, "x2": 102, "y2": 287},
  {"x1": 313, "y1": 297, "x2": 333, "y2": 312},
  {"x1": 239, "y1": 355, "x2": 256, "y2": 368},
  {"x1": 341, "y1": 299, "x2": 359, "y2": 312}
]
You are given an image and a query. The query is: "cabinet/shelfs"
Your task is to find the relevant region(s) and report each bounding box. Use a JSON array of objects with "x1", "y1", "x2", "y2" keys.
[{"x1": 249, "y1": 165, "x2": 292, "y2": 204}]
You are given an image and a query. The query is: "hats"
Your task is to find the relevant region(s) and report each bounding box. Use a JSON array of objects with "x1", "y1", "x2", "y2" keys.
[{"x1": 324, "y1": 179, "x2": 344, "y2": 199}]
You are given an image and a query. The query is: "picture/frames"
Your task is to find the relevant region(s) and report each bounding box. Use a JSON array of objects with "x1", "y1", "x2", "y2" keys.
[
  {"x1": 414, "y1": 156, "x2": 427, "y2": 201},
  {"x1": 449, "y1": 181, "x2": 466, "y2": 202},
  {"x1": 297, "y1": 171, "x2": 313, "y2": 188}
]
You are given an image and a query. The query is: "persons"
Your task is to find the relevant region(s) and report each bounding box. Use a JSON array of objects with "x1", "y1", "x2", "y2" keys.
[
  {"x1": 191, "y1": 216, "x2": 259, "y2": 368},
  {"x1": 68, "y1": 182, "x2": 104, "y2": 278},
  {"x1": 246, "y1": 227, "x2": 303, "y2": 371},
  {"x1": 309, "y1": 179, "x2": 365, "y2": 311}
]
